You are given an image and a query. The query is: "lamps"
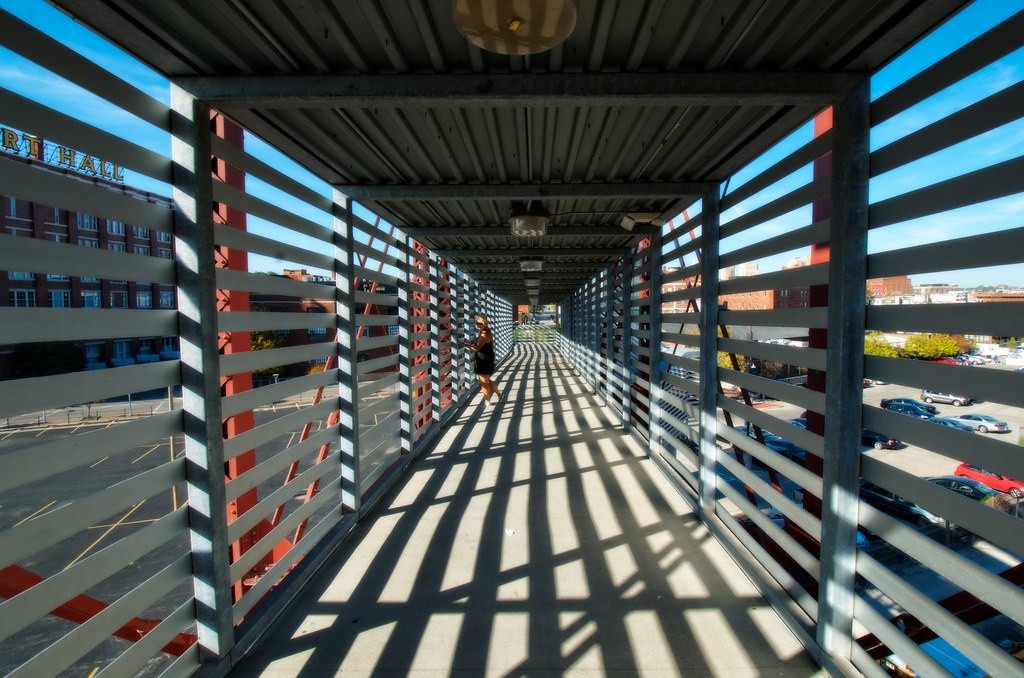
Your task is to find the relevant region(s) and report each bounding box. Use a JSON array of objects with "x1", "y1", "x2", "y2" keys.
[
  {"x1": 452, "y1": 0, "x2": 577, "y2": 56},
  {"x1": 507, "y1": 205, "x2": 550, "y2": 237},
  {"x1": 523, "y1": 277, "x2": 541, "y2": 305},
  {"x1": 518, "y1": 256, "x2": 543, "y2": 271}
]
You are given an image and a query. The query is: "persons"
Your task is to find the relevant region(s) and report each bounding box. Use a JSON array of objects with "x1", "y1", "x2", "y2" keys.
[
  {"x1": 531, "y1": 313, "x2": 554, "y2": 325},
  {"x1": 457, "y1": 314, "x2": 504, "y2": 406}
]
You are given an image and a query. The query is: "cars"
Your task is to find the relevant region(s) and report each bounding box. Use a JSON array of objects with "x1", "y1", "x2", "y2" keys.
[
  {"x1": 918, "y1": 351, "x2": 1009, "y2": 406},
  {"x1": 856, "y1": 532, "x2": 870, "y2": 554},
  {"x1": 921, "y1": 475, "x2": 1004, "y2": 504},
  {"x1": 954, "y1": 463, "x2": 1024, "y2": 500},
  {"x1": 671, "y1": 350, "x2": 700, "y2": 406},
  {"x1": 885, "y1": 401, "x2": 934, "y2": 419},
  {"x1": 733, "y1": 418, "x2": 807, "y2": 460},
  {"x1": 858, "y1": 478, "x2": 949, "y2": 529},
  {"x1": 944, "y1": 412, "x2": 1009, "y2": 434},
  {"x1": 880, "y1": 398, "x2": 938, "y2": 415},
  {"x1": 923, "y1": 415, "x2": 975, "y2": 435},
  {"x1": 862, "y1": 429, "x2": 901, "y2": 450}
]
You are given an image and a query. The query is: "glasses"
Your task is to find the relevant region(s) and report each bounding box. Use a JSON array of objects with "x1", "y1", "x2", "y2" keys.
[{"x1": 476, "y1": 323, "x2": 483, "y2": 325}]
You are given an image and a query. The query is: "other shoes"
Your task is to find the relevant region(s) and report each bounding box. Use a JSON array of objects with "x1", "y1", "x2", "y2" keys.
[
  {"x1": 499, "y1": 394, "x2": 505, "y2": 403},
  {"x1": 482, "y1": 402, "x2": 491, "y2": 406}
]
